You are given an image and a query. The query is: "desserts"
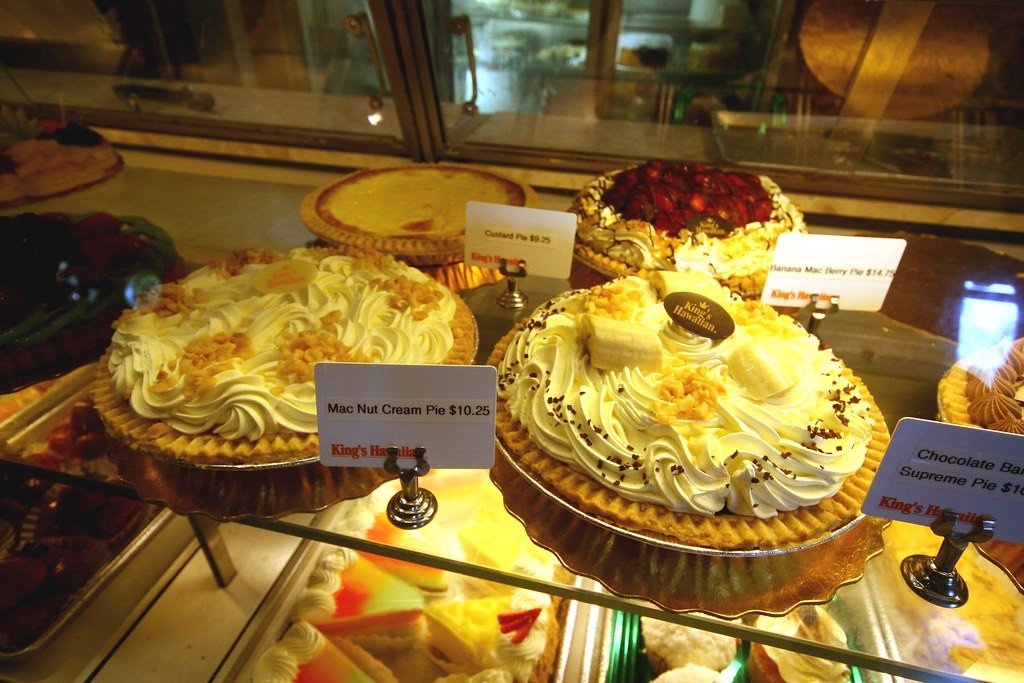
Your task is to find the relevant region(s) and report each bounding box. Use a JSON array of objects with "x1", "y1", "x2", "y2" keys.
[
  {"x1": 254, "y1": 467, "x2": 1024, "y2": 683},
  {"x1": 0, "y1": 401, "x2": 145, "y2": 648}
]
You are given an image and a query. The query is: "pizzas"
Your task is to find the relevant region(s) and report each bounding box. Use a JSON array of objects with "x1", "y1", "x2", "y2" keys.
[{"x1": 299, "y1": 161, "x2": 540, "y2": 254}]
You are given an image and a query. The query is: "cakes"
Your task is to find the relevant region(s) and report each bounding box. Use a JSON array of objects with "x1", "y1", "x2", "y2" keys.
[
  {"x1": 0, "y1": 105, "x2": 118, "y2": 203},
  {"x1": 91, "y1": 244, "x2": 475, "y2": 466},
  {"x1": 482, "y1": 268, "x2": 891, "y2": 554},
  {"x1": 0, "y1": 209, "x2": 187, "y2": 395},
  {"x1": 937, "y1": 334, "x2": 1023, "y2": 436},
  {"x1": 569, "y1": 156, "x2": 808, "y2": 297}
]
